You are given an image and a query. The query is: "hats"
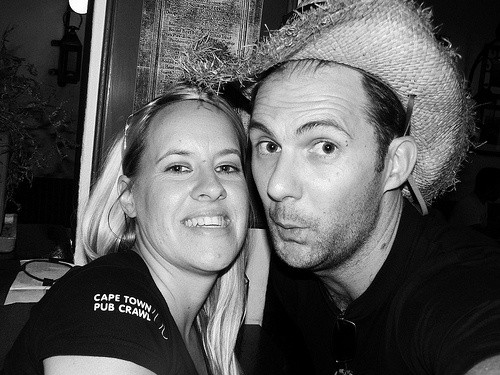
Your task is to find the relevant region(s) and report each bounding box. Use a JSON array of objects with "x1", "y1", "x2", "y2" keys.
[{"x1": 182, "y1": 0, "x2": 474, "y2": 207}]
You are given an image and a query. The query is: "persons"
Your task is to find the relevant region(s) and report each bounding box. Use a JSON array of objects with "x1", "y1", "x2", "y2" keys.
[
  {"x1": 0, "y1": 87, "x2": 270, "y2": 375},
  {"x1": 180, "y1": 0, "x2": 500, "y2": 375}
]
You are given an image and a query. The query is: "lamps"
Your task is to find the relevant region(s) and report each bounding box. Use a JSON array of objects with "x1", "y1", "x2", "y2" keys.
[{"x1": 49, "y1": 0, "x2": 88, "y2": 89}]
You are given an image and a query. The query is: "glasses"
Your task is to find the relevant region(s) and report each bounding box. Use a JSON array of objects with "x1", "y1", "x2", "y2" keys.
[{"x1": 333, "y1": 313, "x2": 357, "y2": 375}]
